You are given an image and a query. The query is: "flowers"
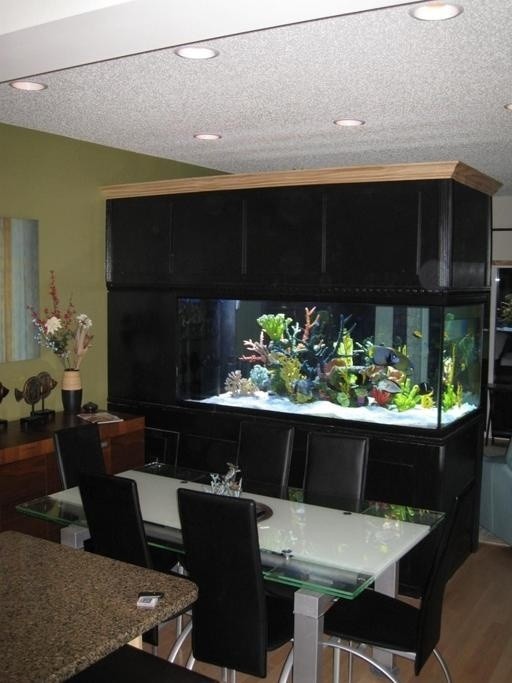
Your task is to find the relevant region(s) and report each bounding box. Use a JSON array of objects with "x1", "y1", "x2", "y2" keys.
[{"x1": 23, "y1": 263, "x2": 96, "y2": 368}]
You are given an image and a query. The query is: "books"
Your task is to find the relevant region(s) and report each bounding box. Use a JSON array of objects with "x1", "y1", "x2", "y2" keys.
[{"x1": 77, "y1": 411, "x2": 124, "y2": 424}]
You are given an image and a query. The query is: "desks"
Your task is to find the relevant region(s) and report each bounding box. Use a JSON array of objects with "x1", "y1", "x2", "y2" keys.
[
  {"x1": 15, "y1": 462, "x2": 451, "y2": 681},
  {"x1": 1, "y1": 528, "x2": 202, "y2": 682}
]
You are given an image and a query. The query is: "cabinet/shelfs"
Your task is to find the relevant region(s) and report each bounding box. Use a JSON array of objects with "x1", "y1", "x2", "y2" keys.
[
  {"x1": 173, "y1": 191, "x2": 244, "y2": 285},
  {"x1": 107, "y1": 286, "x2": 176, "y2": 399},
  {"x1": 325, "y1": 180, "x2": 490, "y2": 286},
  {"x1": 0, "y1": 410, "x2": 147, "y2": 528},
  {"x1": 476, "y1": 265, "x2": 512, "y2": 462},
  {"x1": 105, "y1": 188, "x2": 173, "y2": 286},
  {"x1": 245, "y1": 182, "x2": 324, "y2": 285}
]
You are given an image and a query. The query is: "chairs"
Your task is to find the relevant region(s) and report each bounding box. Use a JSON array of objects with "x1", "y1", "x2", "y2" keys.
[
  {"x1": 168, "y1": 484, "x2": 301, "y2": 683},
  {"x1": 78, "y1": 470, "x2": 185, "y2": 650},
  {"x1": 297, "y1": 427, "x2": 372, "y2": 517},
  {"x1": 273, "y1": 491, "x2": 465, "y2": 682},
  {"x1": 53, "y1": 418, "x2": 105, "y2": 495},
  {"x1": 231, "y1": 416, "x2": 299, "y2": 499}
]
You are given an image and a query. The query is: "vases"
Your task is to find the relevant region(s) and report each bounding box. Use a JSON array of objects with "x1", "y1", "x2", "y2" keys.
[{"x1": 59, "y1": 364, "x2": 87, "y2": 411}]
